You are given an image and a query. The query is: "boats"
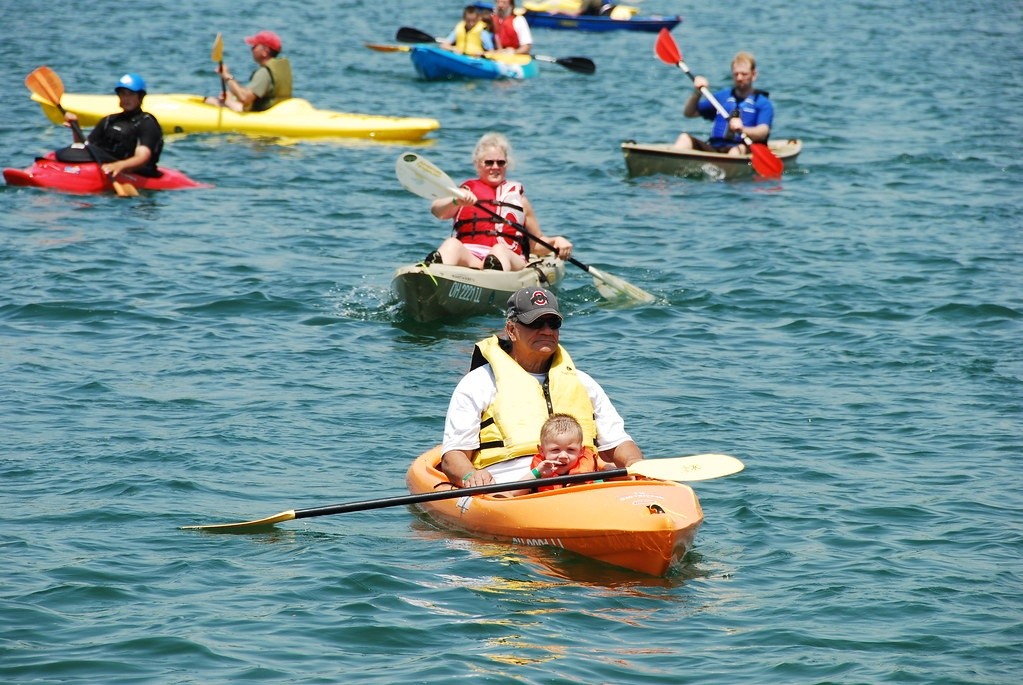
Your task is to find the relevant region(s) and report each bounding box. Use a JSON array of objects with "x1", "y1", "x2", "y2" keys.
[
  {"x1": 406, "y1": 442, "x2": 706, "y2": 579},
  {"x1": 471, "y1": 0, "x2": 686, "y2": 35},
  {"x1": 30, "y1": 91, "x2": 441, "y2": 145},
  {"x1": 619, "y1": 137, "x2": 804, "y2": 185},
  {"x1": 2, "y1": 142, "x2": 214, "y2": 196},
  {"x1": 389, "y1": 243, "x2": 566, "y2": 322},
  {"x1": 408, "y1": 41, "x2": 542, "y2": 81}
]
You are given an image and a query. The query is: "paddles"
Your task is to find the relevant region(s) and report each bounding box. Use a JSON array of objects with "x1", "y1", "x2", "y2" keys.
[
  {"x1": 22, "y1": 63, "x2": 139, "y2": 197},
  {"x1": 395, "y1": 150, "x2": 656, "y2": 304},
  {"x1": 211, "y1": 31, "x2": 227, "y2": 100},
  {"x1": 178, "y1": 453, "x2": 748, "y2": 531},
  {"x1": 397, "y1": 26, "x2": 597, "y2": 76},
  {"x1": 654, "y1": 26, "x2": 785, "y2": 178}
]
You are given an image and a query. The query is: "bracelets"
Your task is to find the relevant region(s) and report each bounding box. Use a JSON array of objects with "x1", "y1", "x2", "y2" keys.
[
  {"x1": 532, "y1": 467, "x2": 541, "y2": 479},
  {"x1": 462, "y1": 471, "x2": 473, "y2": 488},
  {"x1": 452, "y1": 196, "x2": 459, "y2": 207},
  {"x1": 224, "y1": 74, "x2": 234, "y2": 82}
]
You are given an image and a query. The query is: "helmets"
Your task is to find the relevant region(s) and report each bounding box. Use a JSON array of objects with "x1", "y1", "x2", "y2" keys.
[{"x1": 114, "y1": 73, "x2": 148, "y2": 98}]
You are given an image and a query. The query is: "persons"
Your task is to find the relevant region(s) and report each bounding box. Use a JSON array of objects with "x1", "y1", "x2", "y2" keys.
[
  {"x1": 439, "y1": 6, "x2": 494, "y2": 57},
  {"x1": 510, "y1": 413, "x2": 636, "y2": 496},
  {"x1": 674, "y1": 50, "x2": 774, "y2": 155},
  {"x1": 215, "y1": 29, "x2": 292, "y2": 112},
  {"x1": 63, "y1": 71, "x2": 163, "y2": 178},
  {"x1": 579, "y1": 0, "x2": 615, "y2": 16},
  {"x1": 423, "y1": 132, "x2": 573, "y2": 271},
  {"x1": 483, "y1": 0, "x2": 532, "y2": 55},
  {"x1": 441, "y1": 285, "x2": 644, "y2": 494}
]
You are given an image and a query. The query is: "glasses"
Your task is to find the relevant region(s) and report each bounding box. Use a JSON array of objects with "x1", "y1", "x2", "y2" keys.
[
  {"x1": 484, "y1": 160, "x2": 506, "y2": 167},
  {"x1": 518, "y1": 316, "x2": 562, "y2": 330}
]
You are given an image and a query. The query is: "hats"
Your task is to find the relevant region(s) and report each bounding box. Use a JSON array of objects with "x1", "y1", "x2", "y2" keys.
[
  {"x1": 506, "y1": 287, "x2": 564, "y2": 325},
  {"x1": 244, "y1": 31, "x2": 281, "y2": 54}
]
